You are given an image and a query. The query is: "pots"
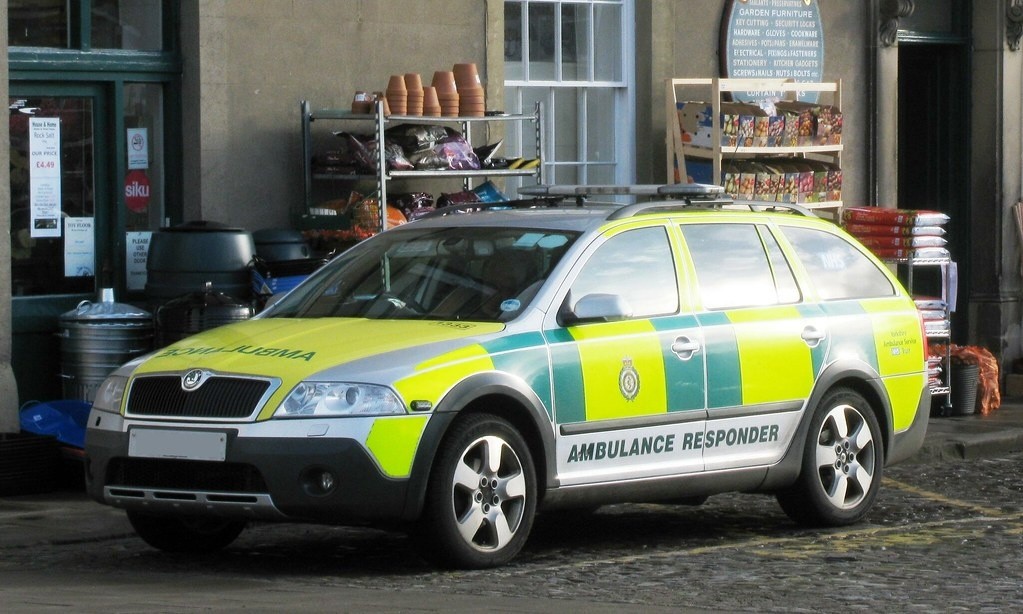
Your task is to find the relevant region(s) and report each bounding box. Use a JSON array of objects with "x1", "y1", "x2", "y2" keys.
[{"x1": 54, "y1": 287, "x2": 156, "y2": 404}]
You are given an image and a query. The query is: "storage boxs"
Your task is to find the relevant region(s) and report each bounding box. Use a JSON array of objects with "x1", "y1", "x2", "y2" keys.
[{"x1": 678, "y1": 100, "x2": 843, "y2": 204}]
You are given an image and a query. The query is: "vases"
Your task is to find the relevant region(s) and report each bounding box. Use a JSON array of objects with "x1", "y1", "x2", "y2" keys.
[{"x1": 937, "y1": 365, "x2": 979, "y2": 415}]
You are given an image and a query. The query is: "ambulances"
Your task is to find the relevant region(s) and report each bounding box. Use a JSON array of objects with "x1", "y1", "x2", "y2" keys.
[{"x1": 86, "y1": 182, "x2": 932, "y2": 576}]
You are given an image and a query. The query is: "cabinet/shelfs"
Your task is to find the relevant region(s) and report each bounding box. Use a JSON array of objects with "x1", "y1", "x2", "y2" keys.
[
  {"x1": 866, "y1": 253, "x2": 955, "y2": 414},
  {"x1": 665, "y1": 77, "x2": 844, "y2": 220},
  {"x1": 300, "y1": 100, "x2": 548, "y2": 298}
]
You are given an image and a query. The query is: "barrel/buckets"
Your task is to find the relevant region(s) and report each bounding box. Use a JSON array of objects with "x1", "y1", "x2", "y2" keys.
[{"x1": 941, "y1": 365, "x2": 980, "y2": 414}]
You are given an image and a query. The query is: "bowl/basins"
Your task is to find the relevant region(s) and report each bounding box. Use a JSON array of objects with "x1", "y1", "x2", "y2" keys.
[{"x1": 352, "y1": 63, "x2": 485, "y2": 118}]
